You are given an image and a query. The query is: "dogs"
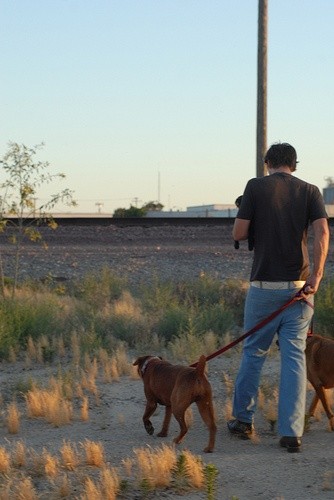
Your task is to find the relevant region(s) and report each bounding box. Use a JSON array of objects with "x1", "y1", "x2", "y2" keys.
[
  {"x1": 132, "y1": 353, "x2": 217, "y2": 455},
  {"x1": 275, "y1": 332, "x2": 334, "y2": 433}
]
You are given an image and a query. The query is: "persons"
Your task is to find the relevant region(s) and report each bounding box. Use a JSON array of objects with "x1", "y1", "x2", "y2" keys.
[{"x1": 226, "y1": 143, "x2": 330, "y2": 452}]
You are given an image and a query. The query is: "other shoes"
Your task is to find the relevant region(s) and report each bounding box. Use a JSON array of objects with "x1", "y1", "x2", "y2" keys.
[
  {"x1": 280, "y1": 435, "x2": 302, "y2": 453},
  {"x1": 226, "y1": 419, "x2": 255, "y2": 440}
]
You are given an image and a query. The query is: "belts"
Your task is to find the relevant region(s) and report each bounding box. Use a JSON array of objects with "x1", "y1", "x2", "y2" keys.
[{"x1": 250, "y1": 280, "x2": 306, "y2": 290}]
